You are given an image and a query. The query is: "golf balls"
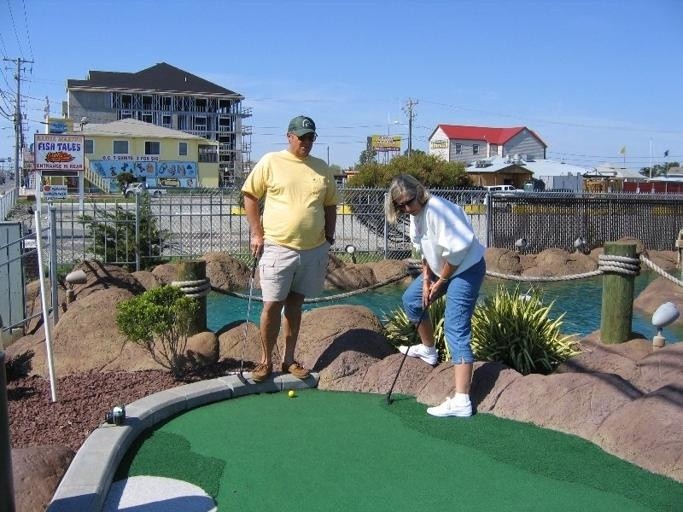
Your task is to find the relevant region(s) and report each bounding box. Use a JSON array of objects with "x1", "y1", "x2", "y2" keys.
[{"x1": 288, "y1": 390, "x2": 294, "y2": 397}]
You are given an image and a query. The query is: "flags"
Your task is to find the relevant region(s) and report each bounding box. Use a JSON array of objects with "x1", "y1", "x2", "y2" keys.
[
  {"x1": 664, "y1": 151, "x2": 668, "y2": 157},
  {"x1": 619, "y1": 146, "x2": 625, "y2": 154}
]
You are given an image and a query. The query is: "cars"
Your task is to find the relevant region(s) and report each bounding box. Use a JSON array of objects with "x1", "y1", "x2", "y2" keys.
[{"x1": 474, "y1": 184, "x2": 523, "y2": 212}]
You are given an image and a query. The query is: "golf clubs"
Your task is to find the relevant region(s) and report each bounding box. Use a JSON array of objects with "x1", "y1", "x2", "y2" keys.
[
  {"x1": 238, "y1": 258, "x2": 257, "y2": 383},
  {"x1": 385, "y1": 300, "x2": 430, "y2": 403}
]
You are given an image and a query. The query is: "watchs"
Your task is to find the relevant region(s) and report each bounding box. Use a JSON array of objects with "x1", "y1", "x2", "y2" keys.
[
  {"x1": 325, "y1": 236, "x2": 335, "y2": 245},
  {"x1": 438, "y1": 276, "x2": 448, "y2": 283}
]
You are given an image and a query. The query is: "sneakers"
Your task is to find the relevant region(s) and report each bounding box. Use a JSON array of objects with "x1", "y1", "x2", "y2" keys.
[
  {"x1": 281, "y1": 358, "x2": 311, "y2": 380},
  {"x1": 398, "y1": 338, "x2": 441, "y2": 369},
  {"x1": 425, "y1": 393, "x2": 473, "y2": 420},
  {"x1": 250, "y1": 362, "x2": 274, "y2": 383}
]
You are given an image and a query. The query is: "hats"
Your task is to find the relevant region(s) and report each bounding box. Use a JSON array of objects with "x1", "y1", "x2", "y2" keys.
[{"x1": 286, "y1": 115, "x2": 319, "y2": 142}]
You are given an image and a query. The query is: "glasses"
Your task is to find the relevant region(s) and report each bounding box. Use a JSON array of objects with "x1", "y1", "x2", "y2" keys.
[
  {"x1": 295, "y1": 133, "x2": 318, "y2": 142},
  {"x1": 392, "y1": 197, "x2": 416, "y2": 208}
]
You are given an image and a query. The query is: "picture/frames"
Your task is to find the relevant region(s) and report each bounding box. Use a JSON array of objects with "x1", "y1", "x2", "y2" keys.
[{"x1": 34, "y1": 134, "x2": 84, "y2": 171}]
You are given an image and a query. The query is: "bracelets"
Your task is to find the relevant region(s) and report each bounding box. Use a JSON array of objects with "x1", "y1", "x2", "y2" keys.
[{"x1": 423, "y1": 278, "x2": 431, "y2": 284}]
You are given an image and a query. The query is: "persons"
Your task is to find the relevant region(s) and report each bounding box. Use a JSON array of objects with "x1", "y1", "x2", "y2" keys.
[
  {"x1": 383, "y1": 173, "x2": 485, "y2": 417},
  {"x1": 240, "y1": 116, "x2": 341, "y2": 383}
]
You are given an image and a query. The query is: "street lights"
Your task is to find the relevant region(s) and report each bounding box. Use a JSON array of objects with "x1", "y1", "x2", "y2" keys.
[
  {"x1": 385, "y1": 120, "x2": 399, "y2": 164},
  {"x1": 1, "y1": 126, "x2": 19, "y2": 198}
]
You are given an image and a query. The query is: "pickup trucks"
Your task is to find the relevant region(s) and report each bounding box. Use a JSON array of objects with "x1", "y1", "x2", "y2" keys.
[{"x1": 123, "y1": 182, "x2": 169, "y2": 197}]
